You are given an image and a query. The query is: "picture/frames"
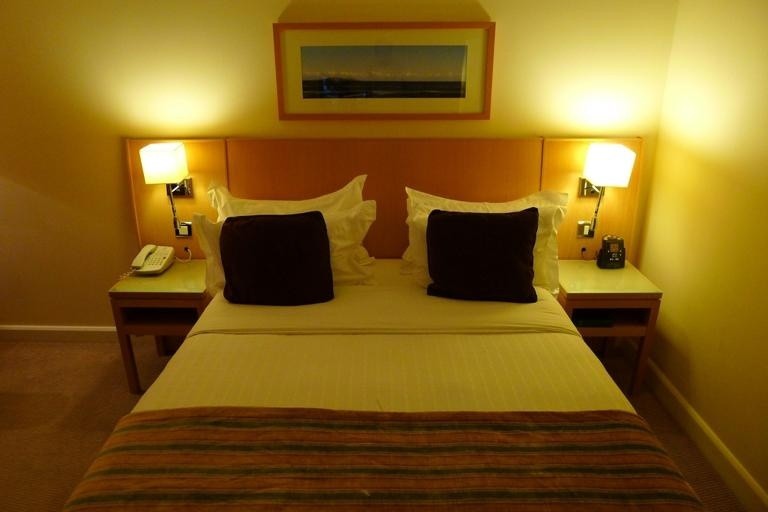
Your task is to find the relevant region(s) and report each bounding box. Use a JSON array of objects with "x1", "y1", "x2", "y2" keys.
[{"x1": 271, "y1": 21, "x2": 496, "y2": 121}]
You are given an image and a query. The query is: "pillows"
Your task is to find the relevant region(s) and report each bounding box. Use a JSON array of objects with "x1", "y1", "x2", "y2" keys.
[
  {"x1": 217, "y1": 210, "x2": 336, "y2": 303},
  {"x1": 400, "y1": 185, "x2": 569, "y2": 297},
  {"x1": 425, "y1": 207, "x2": 541, "y2": 303},
  {"x1": 189, "y1": 198, "x2": 378, "y2": 287},
  {"x1": 207, "y1": 173, "x2": 377, "y2": 267}
]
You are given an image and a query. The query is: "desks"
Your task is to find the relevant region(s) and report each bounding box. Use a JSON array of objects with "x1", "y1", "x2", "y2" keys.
[
  {"x1": 556, "y1": 256, "x2": 662, "y2": 400},
  {"x1": 110, "y1": 258, "x2": 214, "y2": 394}
]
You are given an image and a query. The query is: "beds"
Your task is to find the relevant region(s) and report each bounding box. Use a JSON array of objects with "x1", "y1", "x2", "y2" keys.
[{"x1": 60, "y1": 257, "x2": 709, "y2": 512}]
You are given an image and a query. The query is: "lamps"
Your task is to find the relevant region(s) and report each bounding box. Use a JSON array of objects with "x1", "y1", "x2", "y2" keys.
[
  {"x1": 576, "y1": 142, "x2": 637, "y2": 238},
  {"x1": 139, "y1": 141, "x2": 194, "y2": 236}
]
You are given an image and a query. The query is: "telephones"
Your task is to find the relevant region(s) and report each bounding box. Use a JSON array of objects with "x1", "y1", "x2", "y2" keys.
[{"x1": 131, "y1": 244, "x2": 175, "y2": 274}]
